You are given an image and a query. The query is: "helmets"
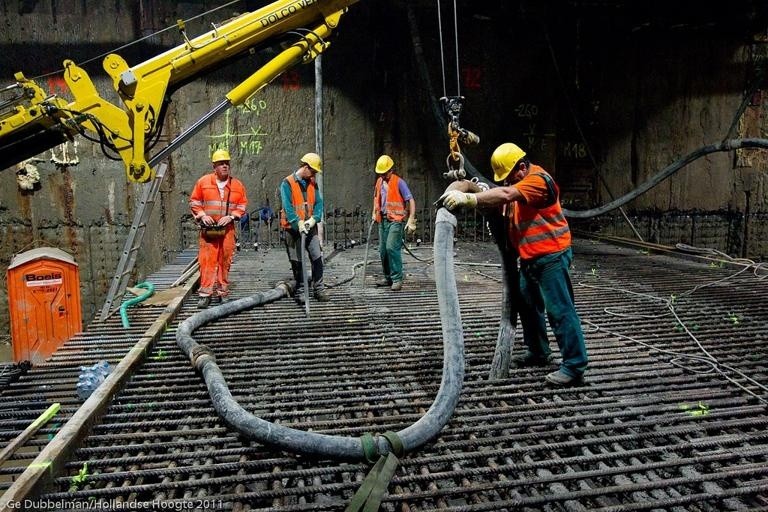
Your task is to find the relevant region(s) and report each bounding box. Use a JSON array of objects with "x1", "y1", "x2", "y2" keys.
[
  {"x1": 491, "y1": 142, "x2": 526, "y2": 182},
  {"x1": 212, "y1": 149, "x2": 231, "y2": 163},
  {"x1": 375, "y1": 154, "x2": 394, "y2": 174},
  {"x1": 300, "y1": 153, "x2": 324, "y2": 173}
]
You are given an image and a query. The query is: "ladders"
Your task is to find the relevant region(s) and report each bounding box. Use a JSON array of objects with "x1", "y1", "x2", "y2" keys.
[{"x1": 102, "y1": 162, "x2": 168, "y2": 316}]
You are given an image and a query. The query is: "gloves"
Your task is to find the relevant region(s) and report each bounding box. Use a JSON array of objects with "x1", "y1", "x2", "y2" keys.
[
  {"x1": 304, "y1": 217, "x2": 316, "y2": 229},
  {"x1": 403, "y1": 218, "x2": 417, "y2": 236},
  {"x1": 439, "y1": 189, "x2": 478, "y2": 211},
  {"x1": 298, "y1": 220, "x2": 309, "y2": 235}
]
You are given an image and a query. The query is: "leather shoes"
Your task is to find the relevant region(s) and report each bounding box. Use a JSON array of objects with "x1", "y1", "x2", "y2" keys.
[
  {"x1": 511, "y1": 353, "x2": 547, "y2": 364},
  {"x1": 315, "y1": 290, "x2": 330, "y2": 302},
  {"x1": 219, "y1": 295, "x2": 229, "y2": 305},
  {"x1": 391, "y1": 281, "x2": 401, "y2": 290},
  {"x1": 376, "y1": 278, "x2": 392, "y2": 286},
  {"x1": 197, "y1": 297, "x2": 211, "y2": 309},
  {"x1": 298, "y1": 292, "x2": 306, "y2": 305},
  {"x1": 545, "y1": 368, "x2": 585, "y2": 386}
]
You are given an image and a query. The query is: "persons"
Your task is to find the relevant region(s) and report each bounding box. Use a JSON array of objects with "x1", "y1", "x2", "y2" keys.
[
  {"x1": 189, "y1": 150, "x2": 248, "y2": 308},
  {"x1": 371, "y1": 156, "x2": 417, "y2": 291},
  {"x1": 437, "y1": 143, "x2": 588, "y2": 385},
  {"x1": 279, "y1": 150, "x2": 332, "y2": 303}
]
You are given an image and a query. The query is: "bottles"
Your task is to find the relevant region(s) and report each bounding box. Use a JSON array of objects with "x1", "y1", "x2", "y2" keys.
[{"x1": 75, "y1": 359, "x2": 111, "y2": 401}]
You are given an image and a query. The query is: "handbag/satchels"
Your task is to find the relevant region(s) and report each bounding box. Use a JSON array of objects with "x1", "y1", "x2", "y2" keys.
[{"x1": 205, "y1": 222, "x2": 227, "y2": 238}]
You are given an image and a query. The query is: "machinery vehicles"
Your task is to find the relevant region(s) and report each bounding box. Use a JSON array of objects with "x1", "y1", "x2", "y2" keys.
[{"x1": 2, "y1": 0, "x2": 479, "y2": 184}]
